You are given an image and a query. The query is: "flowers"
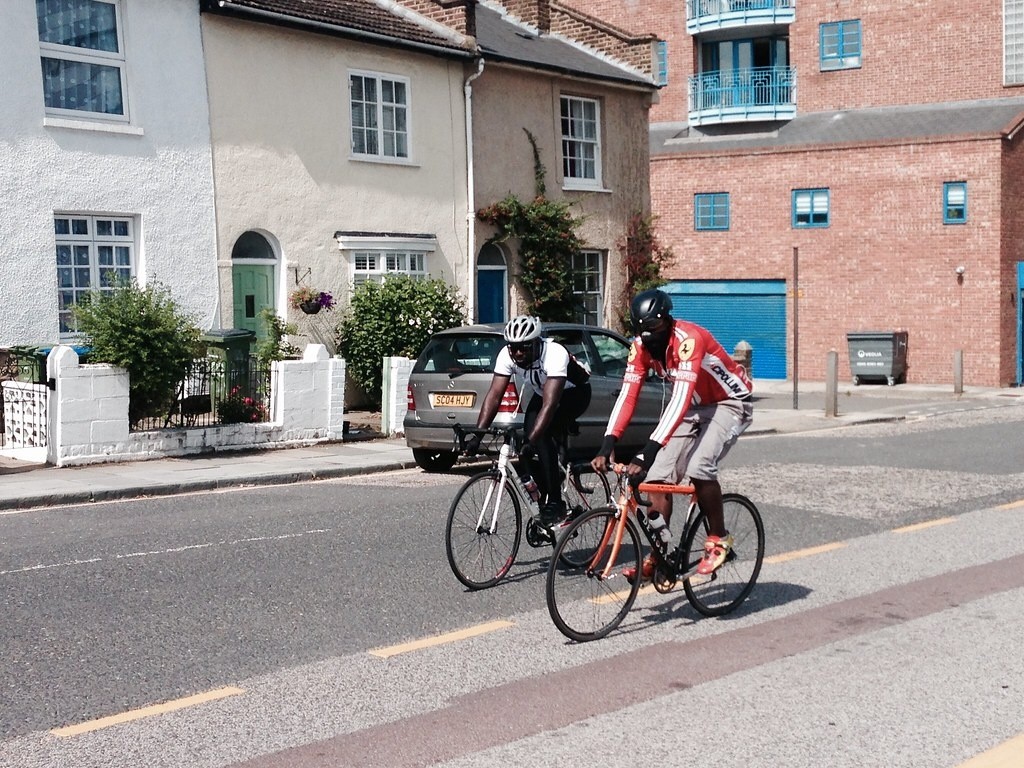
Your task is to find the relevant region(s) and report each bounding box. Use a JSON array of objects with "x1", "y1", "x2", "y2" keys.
[{"x1": 286, "y1": 286, "x2": 337, "y2": 313}]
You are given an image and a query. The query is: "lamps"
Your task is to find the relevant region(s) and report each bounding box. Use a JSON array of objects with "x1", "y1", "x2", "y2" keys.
[{"x1": 955, "y1": 266, "x2": 965, "y2": 274}]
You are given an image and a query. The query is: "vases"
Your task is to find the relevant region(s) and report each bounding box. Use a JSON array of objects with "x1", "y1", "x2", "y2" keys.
[{"x1": 299, "y1": 303, "x2": 322, "y2": 314}]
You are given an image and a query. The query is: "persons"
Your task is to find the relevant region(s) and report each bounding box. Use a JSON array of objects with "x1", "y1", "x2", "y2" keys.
[
  {"x1": 465, "y1": 315, "x2": 593, "y2": 531},
  {"x1": 591, "y1": 290, "x2": 753, "y2": 580}
]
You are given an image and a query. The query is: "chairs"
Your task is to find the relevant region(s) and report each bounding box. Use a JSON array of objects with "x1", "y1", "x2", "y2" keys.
[{"x1": 433, "y1": 350, "x2": 464, "y2": 372}]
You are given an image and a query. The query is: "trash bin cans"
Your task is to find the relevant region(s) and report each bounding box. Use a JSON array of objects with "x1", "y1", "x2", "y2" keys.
[
  {"x1": 14, "y1": 341, "x2": 93, "y2": 385},
  {"x1": 202, "y1": 325, "x2": 257, "y2": 417},
  {"x1": 846, "y1": 329, "x2": 908, "y2": 384}
]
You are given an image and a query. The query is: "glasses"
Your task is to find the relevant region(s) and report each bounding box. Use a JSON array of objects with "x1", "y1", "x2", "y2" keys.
[
  {"x1": 633, "y1": 316, "x2": 669, "y2": 334},
  {"x1": 506, "y1": 343, "x2": 535, "y2": 351}
]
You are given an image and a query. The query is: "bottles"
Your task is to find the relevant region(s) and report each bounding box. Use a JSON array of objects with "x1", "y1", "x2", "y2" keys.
[
  {"x1": 647, "y1": 510, "x2": 671, "y2": 543},
  {"x1": 521, "y1": 474, "x2": 540, "y2": 501}
]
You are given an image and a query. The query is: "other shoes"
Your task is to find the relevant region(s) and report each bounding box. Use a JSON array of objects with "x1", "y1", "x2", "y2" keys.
[{"x1": 541, "y1": 499, "x2": 567, "y2": 520}]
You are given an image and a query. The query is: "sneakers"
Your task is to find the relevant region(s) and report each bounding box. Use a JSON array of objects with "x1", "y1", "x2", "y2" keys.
[
  {"x1": 698, "y1": 529, "x2": 734, "y2": 572},
  {"x1": 623, "y1": 555, "x2": 658, "y2": 580}
]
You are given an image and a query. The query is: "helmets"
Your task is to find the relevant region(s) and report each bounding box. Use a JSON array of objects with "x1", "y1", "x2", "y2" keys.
[
  {"x1": 504, "y1": 315, "x2": 544, "y2": 343},
  {"x1": 629, "y1": 290, "x2": 673, "y2": 326}
]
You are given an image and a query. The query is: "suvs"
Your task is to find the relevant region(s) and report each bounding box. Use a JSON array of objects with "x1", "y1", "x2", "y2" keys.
[{"x1": 403, "y1": 320, "x2": 676, "y2": 473}]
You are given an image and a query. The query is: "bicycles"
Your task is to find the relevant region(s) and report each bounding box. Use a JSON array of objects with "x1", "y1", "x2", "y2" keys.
[
  {"x1": 545, "y1": 463, "x2": 766, "y2": 643},
  {"x1": 445, "y1": 422, "x2": 613, "y2": 591}
]
